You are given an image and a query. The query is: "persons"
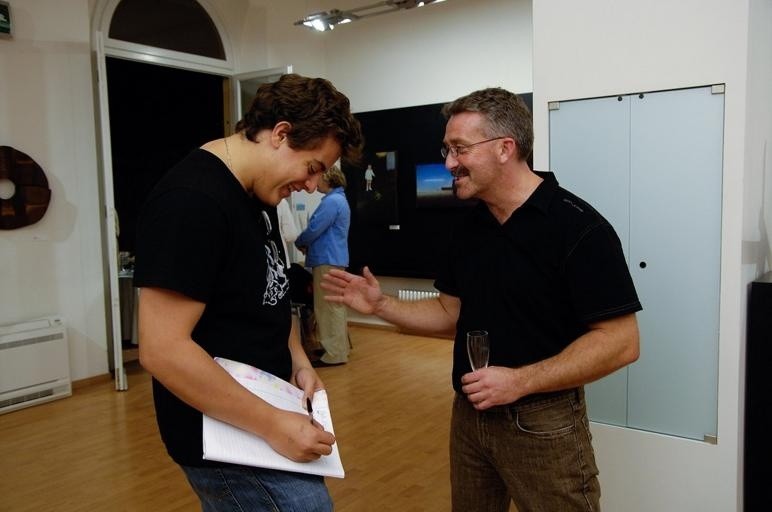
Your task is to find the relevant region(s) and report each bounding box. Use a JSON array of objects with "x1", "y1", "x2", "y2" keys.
[
  {"x1": 274, "y1": 193, "x2": 301, "y2": 270},
  {"x1": 364, "y1": 161, "x2": 375, "y2": 192},
  {"x1": 319, "y1": 87, "x2": 642, "y2": 511},
  {"x1": 296, "y1": 163, "x2": 357, "y2": 369},
  {"x1": 131, "y1": 72, "x2": 369, "y2": 511}
]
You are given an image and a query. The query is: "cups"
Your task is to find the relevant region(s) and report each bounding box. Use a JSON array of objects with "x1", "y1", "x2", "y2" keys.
[{"x1": 466, "y1": 329, "x2": 491, "y2": 372}]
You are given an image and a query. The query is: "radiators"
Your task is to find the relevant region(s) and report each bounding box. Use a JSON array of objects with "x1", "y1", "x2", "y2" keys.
[
  {"x1": 397, "y1": 290, "x2": 455, "y2": 339},
  {"x1": 0, "y1": 314, "x2": 72, "y2": 415}
]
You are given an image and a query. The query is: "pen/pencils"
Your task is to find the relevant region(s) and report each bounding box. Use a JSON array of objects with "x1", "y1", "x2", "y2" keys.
[{"x1": 307, "y1": 398, "x2": 317, "y2": 426}]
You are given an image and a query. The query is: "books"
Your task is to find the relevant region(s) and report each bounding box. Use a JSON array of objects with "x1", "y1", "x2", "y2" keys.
[{"x1": 197, "y1": 353, "x2": 349, "y2": 480}]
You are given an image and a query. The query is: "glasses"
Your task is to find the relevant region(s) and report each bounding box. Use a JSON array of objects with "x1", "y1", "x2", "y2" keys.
[{"x1": 440, "y1": 135, "x2": 507, "y2": 158}]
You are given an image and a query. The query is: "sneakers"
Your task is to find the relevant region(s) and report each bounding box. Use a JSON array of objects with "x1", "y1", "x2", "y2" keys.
[{"x1": 311, "y1": 347, "x2": 347, "y2": 368}]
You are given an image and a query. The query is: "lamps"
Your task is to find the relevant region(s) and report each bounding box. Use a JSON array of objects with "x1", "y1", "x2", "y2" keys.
[{"x1": 294, "y1": 0, "x2": 446, "y2": 33}]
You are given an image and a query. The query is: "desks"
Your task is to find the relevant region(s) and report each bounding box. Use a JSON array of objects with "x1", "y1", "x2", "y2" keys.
[{"x1": 116, "y1": 270, "x2": 142, "y2": 345}]
